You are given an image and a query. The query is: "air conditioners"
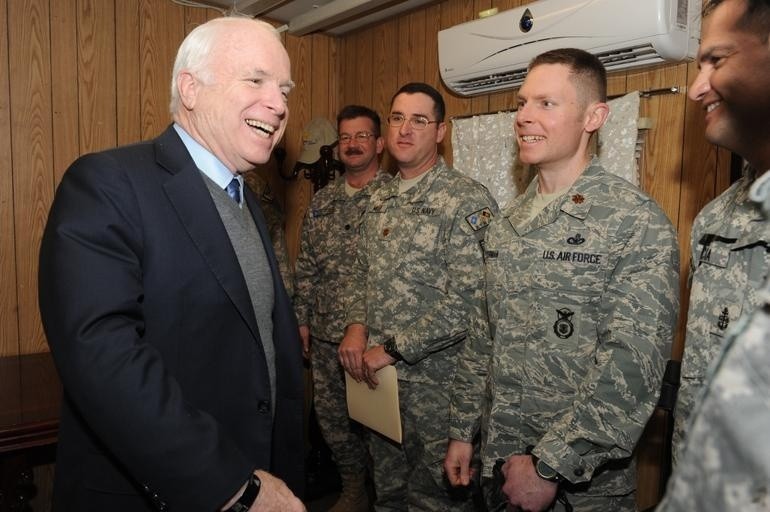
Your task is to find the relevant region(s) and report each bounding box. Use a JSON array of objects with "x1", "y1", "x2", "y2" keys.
[{"x1": 438, "y1": 0, "x2": 702, "y2": 98}]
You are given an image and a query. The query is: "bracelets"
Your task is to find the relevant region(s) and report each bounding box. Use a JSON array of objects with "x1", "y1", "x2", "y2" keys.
[{"x1": 224, "y1": 475, "x2": 261, "y2": 512}]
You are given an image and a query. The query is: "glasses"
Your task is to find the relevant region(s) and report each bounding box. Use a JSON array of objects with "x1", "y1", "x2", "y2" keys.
[
  {"x1": 388, "y1": 114, "x2": 441, "y2": 130},
  {"x1": 339, "y1": 132, "x2": 377, "y2": 143}
]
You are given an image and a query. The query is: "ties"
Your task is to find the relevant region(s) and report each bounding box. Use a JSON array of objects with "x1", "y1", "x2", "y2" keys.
[{"x1": 228, "y1": 178, "x2": 241, "y2": 204}]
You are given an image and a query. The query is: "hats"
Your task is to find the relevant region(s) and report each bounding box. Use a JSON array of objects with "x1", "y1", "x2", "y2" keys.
[{"x1": 297, "y1": 118, "x2": 338, "y2": 165}]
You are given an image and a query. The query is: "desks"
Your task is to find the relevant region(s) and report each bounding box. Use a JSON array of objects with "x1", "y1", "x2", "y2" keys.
[{"x1": 2, "y1": 351, "x2": 67, "y2": 510}]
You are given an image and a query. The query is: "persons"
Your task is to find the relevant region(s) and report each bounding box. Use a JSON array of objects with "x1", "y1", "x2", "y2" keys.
[
  {"x1": 38, "y1": 17, "x2": 311, "y2": 512},
  {"x1": 239, "y1": 171, "x2": 296, "y2": 301},
  {"x1": 337, "y1": 82, "x2": 498, "y2": 512},
  {"x1": 444, "y1": 49, "x2": 678, "y2": 512},
  {"x1": 295, "y1": 105, "x2": 393, "y2": 512},
  {"x1": 655, "y1": 0, "x2": 770, "y2": 512}
]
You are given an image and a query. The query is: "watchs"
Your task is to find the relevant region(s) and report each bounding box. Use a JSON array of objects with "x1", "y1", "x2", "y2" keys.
[
  {"x1": 531, "y1": 456, "x2": 561, "y2": 482},
  {"x1": 384, "y1": 338, "x2": 404, "y2": 361}
]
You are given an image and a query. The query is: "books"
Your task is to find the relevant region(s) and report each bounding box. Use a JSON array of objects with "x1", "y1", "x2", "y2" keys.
[{"x1": 345, "y1": 364, "x2": 403, "y2": 445}]
